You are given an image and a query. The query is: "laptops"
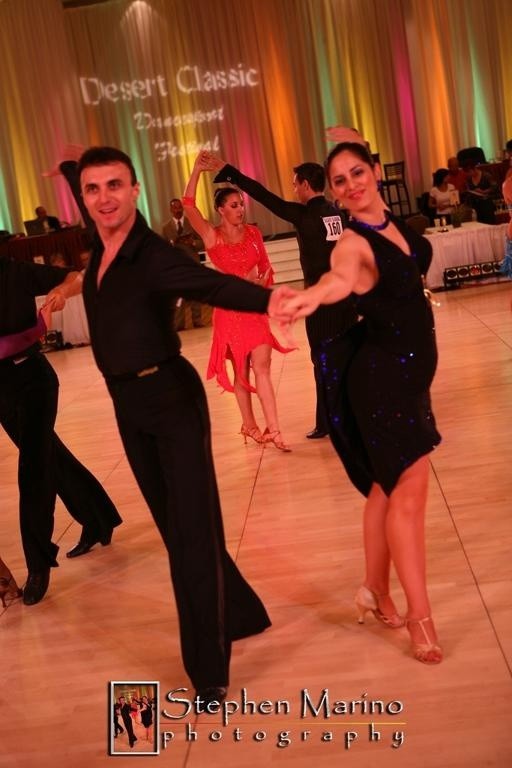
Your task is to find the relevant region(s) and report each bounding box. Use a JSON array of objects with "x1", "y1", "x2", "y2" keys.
[{"x1": 24, "y1": 220, "x2": 46, "y2": 236}]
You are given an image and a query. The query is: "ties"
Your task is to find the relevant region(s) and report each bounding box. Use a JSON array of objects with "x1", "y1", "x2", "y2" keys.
[{"x1": 177, "y1": 221, "x2": 183, "y2": 233}]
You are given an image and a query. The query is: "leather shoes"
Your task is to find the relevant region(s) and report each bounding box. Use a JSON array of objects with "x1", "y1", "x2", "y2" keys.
[
  {"x1": 23, "y1": 568, "x2": 51, "y2": 605},
  {"x1": 67, "y1": 528, "x2": 113, "y2": 557},
  {"x1": 193, "y1": 688, "x2": 227, "y2": 712},
  {"x1": 306, "y1": 427, "x2": 329, "y2": 438}
]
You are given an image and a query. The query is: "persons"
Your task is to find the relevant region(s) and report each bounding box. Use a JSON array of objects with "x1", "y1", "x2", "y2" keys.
[
  {"x1": 38, "y1": 144, "x2": 305, "y2": 710},
  {"x1": 2, "y1": 294, "x2": 60, "y2": 610},
  {"x1": 1, "y1": 139, "x2": 511, "y2": 459},
  {"x1": 110, "y1": 688, "x2": 155, "y2": 751},
  {"x1": 2, "y1": 226, "x2": 122, "y2": 609},
  {"x1": 272, "y1": 121, "x2": 445, "y2": 669}
]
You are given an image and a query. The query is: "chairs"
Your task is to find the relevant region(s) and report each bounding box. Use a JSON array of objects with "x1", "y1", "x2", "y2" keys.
[
  {"x1": 381, "y1": 163, "x2": 412, "y2": 218},
  {"x1": 404, "y1": 215, "x2": 429, "y2": 234}
]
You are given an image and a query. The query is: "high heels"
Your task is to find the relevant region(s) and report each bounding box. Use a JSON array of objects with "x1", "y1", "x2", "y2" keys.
[
  {"x1": 241, "y1": 423, "x2": 270, "y2": 444},
  {"x1": 262, "y1": 428, "x2": 292, "y2": 452},
  {"x1": 0, "y1": 575, "x2": 23, "y2": 607},
  {"x1": 355, "y1": 585, "x2": 406, "y2": 628},
  {"x1": 404, "y1": 613, "x2": 443, "y2": 665}
]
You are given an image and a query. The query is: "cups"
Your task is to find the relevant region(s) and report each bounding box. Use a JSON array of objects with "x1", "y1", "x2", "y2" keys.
[{"x1": 434, "y1": 216, "x2": 447, "y2": 232}]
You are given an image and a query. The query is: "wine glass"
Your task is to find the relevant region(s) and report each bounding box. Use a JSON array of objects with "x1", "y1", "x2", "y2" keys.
[{"x1": 494, "y1": 198, "x2": 508, "y2": 213}]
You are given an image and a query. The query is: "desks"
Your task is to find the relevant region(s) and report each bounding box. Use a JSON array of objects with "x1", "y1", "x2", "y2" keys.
[
  {"x1": 6, "y1": 225, "x2": 84, "y2": 272},
  {"x1": 423, "y1": 221, "x2": 508, "y2": 290},
  {"x1": 35, "y1": 294, "x2": 90, "y2": 347}
]
